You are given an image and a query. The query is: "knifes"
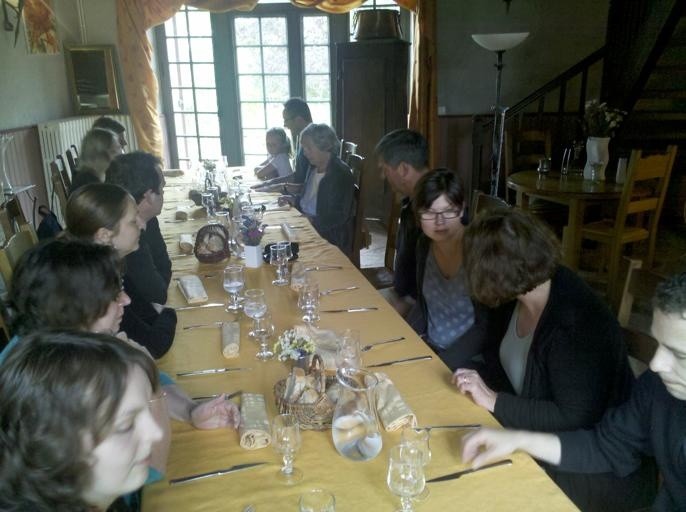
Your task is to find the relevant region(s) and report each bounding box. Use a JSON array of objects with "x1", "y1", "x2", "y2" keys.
[
  {"x1": 405, "y1": 423, "x2": 482, "y2": 434},
  {"x1": 165, "y1": 455, "x2": 271, "y2": 486},
  {"x1": 322, "y1": 306, "x2": 378, "y2": 313},
  {"x1": 423, "y1": 457, "x2": 513, "y2": 484},
  {"x1": 368, "y1": 354, "x2": 433, "y2": 367},
  {"x1": 184, "y1": 318, "x2": 238, "y2": 330}
]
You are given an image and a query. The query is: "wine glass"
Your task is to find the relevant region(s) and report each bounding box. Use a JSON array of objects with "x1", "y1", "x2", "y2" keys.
[
  {"x1": 275, "y1": 413, "x2": 306, "y2": 482},
  {"x1": 387, "y1": 445, "x2": 427, "y2": 512},
  {"x1": 301, "y1": 490, "x2": 334, "y2": 511},
  {"x1": 173, "y1": 154, "x2": 348, "y2": 361}
]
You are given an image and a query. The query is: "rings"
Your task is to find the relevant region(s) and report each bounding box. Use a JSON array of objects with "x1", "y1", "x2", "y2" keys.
[{"x1": 463, "y1": 375, "x2": 467, "y2": 384}]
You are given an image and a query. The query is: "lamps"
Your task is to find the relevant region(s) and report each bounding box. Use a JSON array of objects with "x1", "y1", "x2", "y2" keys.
[{"x1": 470, "y1": 31, "x2": 533, "y2": 193}]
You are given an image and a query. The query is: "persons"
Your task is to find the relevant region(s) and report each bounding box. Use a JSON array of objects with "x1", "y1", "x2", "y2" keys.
[
  {"x1": 91, "y1": 117, "x2": 128, "y2": 152},
  {"x1": 0, "y1": 241, "x2": 245, "y2": 503},
  {"x1": 409, "y1": 167, "x2": 495, "y2": 371},
  {"x1": 253, "y1": 126, "x2": 293, "y2": 179},
  {"x1": 0, "y1": 327, "x2": 165, "y2": 510},
  {"x1": 449, "y1": 205, "x2": 637, "y2": 431},
  {"x1": 460, "y1": 261, "x2": 686, "y2": 512},
  {"x1": 249, "y1": 97, "x2": 314, "y2": 195},
  {"x1": 372, "y1": 128, "x2": 432, "y2": 318},
  {"x1": 68, "y1": 127, "x2": 123, "y2": 195},
  {"x1": 103, "y1": 150, "x2": 172, "y2": 305},
  {"x1": 276, "y1": 124, "x2": 354, "y2": 248},
  {"x1": 53, "y1": 181, "x2": 178, "y2": 360}
]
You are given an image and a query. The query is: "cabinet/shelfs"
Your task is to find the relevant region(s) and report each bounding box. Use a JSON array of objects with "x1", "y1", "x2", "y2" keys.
[{"x1": 332, "y1": 40, "x2": 418, "y2": 235}]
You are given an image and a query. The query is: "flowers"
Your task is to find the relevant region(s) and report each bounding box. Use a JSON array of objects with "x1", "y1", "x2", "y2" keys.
[{"x1": 579, "y1": 98, "x2": 629, "y2": 139}]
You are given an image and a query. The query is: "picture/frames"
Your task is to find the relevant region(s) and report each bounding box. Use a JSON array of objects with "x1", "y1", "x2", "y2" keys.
[{"x1": 20, "y1": 0, "x2": 60, "y2": 57}]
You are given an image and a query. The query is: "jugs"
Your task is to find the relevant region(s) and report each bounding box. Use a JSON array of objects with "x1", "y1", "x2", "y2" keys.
[{"x1": 331, "y1": 366, "x2": 382, "y2": 461}]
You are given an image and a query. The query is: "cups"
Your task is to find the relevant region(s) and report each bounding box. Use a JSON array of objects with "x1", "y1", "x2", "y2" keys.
[{"x1": 536, "y1": 157, "x2": 551, "y2": 176}]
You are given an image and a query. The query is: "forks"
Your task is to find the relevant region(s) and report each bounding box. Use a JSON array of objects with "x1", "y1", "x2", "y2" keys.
[
  {"x1": 362, "y1": 336, "x2": 405, "y2": 352},
  {"x1": 177, "y1": 366, "x2": 242, "y2": 376},
  {"x1": 192, "y1": 390, "x2": 243, "y2": 400},
  {"x1": 319, "y1": 286, "x2": 358, "y2": 297}
]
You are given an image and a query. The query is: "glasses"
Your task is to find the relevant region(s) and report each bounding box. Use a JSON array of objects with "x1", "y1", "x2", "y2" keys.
[{"x1": 417, "y1": 209, "x2": 462, "y2": 221}]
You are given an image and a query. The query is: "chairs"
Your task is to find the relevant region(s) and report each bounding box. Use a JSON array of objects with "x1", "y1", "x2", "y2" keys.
[{"x1": 0, "y1": 135, "x2": 679, "y2": 511}]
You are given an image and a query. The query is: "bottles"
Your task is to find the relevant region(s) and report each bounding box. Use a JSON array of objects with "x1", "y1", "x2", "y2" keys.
[{"x1": 614, "y1": 158, "x2": 628, "y2": 184}]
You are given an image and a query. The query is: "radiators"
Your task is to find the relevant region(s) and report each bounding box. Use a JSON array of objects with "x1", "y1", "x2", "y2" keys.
[{"x1": 38, "y1": 113, "x2": 139, "y2": 222}]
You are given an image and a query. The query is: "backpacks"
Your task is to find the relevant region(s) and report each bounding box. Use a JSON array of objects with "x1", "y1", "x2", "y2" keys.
[{"x1": 34, "y1": 205, "x2": 66, "y2": 238}]
